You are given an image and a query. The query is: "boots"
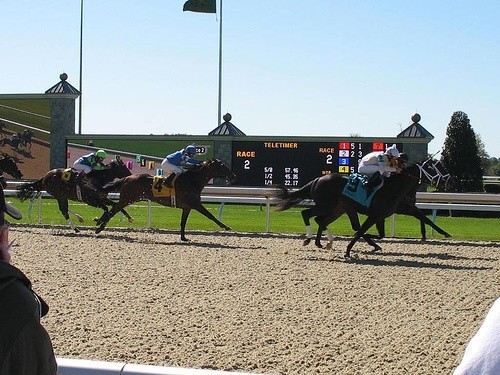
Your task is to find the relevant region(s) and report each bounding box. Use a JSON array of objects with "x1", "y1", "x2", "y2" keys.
[
  {"x1": 165, "y1": 173, "x2": 176, "y2": 187},
  {"x1": 365, "y1": 172, "x2": 382, "y2": 192},
  {"x1": 87, "y1": 170, "x2": 100, "y2": 187}
]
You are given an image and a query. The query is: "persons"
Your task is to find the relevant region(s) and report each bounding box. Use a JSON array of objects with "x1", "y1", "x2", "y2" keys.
[
  {"x1": 73, "y1": 150, "x2": 107, "y2": 189},
  {"x1": 161, "y1": 146, "x2": 204, "y2": 189},
  {"x1": 453, "y1": 298, "x2": 500, "y2": 375},
  {"x1": 357, "y1": 144, "x2": 400, "y2": 187},
  {"x1": 0, "y1": 186, "x2": 57, "y2": 375}
]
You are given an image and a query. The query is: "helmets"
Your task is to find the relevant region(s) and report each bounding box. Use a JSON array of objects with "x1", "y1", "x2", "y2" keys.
[
  {"x1": 185, "y1": 145, "x2": 196, "y2": 154},
  {"x1": 385, "y1": 147, "x2": 398, "y2": 158},
  {"x1": 96, "y1": 150, "x2": 107, "y2": 158}
]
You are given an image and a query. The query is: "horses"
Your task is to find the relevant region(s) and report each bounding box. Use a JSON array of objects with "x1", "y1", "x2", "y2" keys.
[
  {"x1": 0, "y1": 147, "x2": 25, "y2": 189},
  {"x1": 0, "y1": 120, "x2": 36, "y2": 150},
  {"x1": 95, "y1": 156, "x2": 239, "y2": 241},
  {"x1": 267, "y1": 158, "x2": 453, "y2": 258},
  {"x1": 19, "y1": 153, "x2": 135, "y2": 234}
]
